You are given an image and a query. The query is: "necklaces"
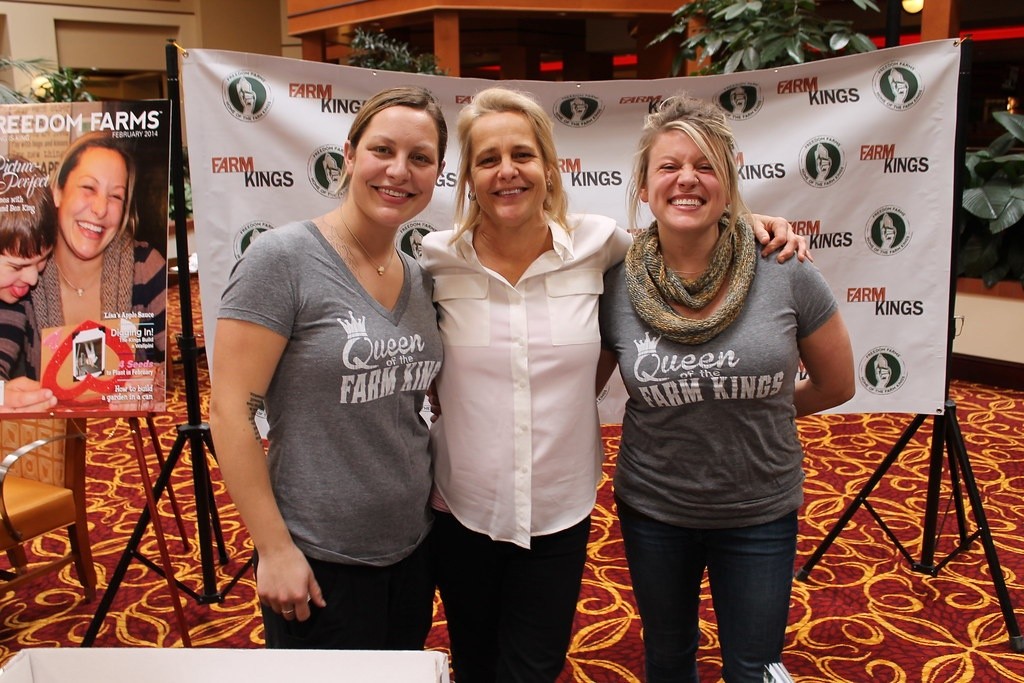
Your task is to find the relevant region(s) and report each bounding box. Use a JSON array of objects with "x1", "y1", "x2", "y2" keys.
[
  {"x1": 341, "y1": 211, "x2": 395, "y2": 275},
  {"x1": 52, "y1": 246, "x2": 101, "y2": 297}
]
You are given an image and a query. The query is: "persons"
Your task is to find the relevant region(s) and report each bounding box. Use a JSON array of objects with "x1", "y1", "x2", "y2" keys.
[
  {"x1": 1, "y1": 133, "x2": 167, "y2": 413},
  {"x1": 208, "y1": 86, "x2": 448, "y2": 650},
  {"x1": 418, "y1": 89, "x2": 814, "y2": 683},
  {"x1": 0, "y1": 153, "x2": 59, "y2": 310},
  {"x1": 596, "y1": 95, "x2": 855, "y2": 683}
]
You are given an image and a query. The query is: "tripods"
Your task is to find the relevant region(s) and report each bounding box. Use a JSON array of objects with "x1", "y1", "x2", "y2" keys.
[
  {"x1": 78, "y1": 42, "x2": 253, "y2": 647},
  {"x1": 795, "y1": 39, "x2": 1024, "y2": 654}
]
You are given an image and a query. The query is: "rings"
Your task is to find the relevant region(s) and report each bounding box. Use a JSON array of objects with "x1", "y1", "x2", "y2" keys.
[
  {"x1": 281, "y1": 609, "x2": 294, "y2": 615},
  {"x1": 12, "y1": 408, "x2": 16, "y2": 413}
]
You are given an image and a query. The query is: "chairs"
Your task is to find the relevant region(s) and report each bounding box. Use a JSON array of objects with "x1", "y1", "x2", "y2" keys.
[{"x1": 0, "y1": 417, "x2": 96, "y2": 603}]
